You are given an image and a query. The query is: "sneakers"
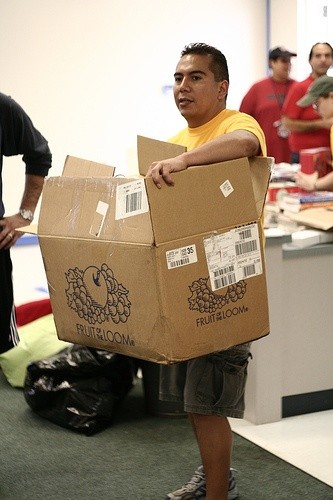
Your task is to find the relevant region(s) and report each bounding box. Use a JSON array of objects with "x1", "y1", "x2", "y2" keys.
[{"x1": 167, "y1": 463, "x2": 238, "y2": 500}]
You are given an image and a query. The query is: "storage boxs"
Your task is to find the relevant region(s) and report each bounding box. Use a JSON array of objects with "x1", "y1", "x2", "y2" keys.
[
  {"x1": 14, "y1": 135, "x2": 275, "y2": 365},
  {"x1": 299, "y1": 148, "x2": 330, "y2": 181}
]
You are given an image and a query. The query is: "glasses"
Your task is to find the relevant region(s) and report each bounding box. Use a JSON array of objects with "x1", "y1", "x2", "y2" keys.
[{"x1": 312, "y1": 103, "x2": 318, "y2": 110}]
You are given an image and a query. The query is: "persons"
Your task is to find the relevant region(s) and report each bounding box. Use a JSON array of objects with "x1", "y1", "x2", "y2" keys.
[
  {"x1": 282, "y1": 42, "x2": 333, "y2": 164},
  {"x1": 0, "y1": 93, "x2": 53, "y2": 356},
  {"x1": 240, "y1": 46, "x2": 301, "y2": 165},
  {"x1": 145, "y1": 42, "x2": 268, "y2": 500},
  {"x1": 294, "y1": 74, "x2": 333, "y2": 193}
]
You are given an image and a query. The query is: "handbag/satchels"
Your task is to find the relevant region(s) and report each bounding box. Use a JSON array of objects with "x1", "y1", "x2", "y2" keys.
[{"x1": 23, "y1": 343, "x2": 136, "y2": 437}]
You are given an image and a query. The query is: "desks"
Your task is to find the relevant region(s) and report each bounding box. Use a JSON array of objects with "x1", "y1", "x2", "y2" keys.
[{"x1": 241, "y1": 191, "x2": 332, "y2": 427}]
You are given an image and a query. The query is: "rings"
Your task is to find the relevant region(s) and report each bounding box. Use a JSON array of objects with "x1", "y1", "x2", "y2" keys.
[{"x1": 8, "y1": 233, "x2": 13, "y2": 239}]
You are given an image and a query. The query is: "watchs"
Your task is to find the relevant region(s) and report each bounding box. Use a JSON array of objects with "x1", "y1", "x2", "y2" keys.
[{"x1": 18, "y1": 208, "x2": 34, "y2": 221}]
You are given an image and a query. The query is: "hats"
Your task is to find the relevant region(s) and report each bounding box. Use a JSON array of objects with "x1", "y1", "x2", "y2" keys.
[
  {"x1": 295, "y1": 76, "x2": 333, "y2": 107},
  {"x1": 268, "y1": 48, "x2": 297, "y2": 61}
]
